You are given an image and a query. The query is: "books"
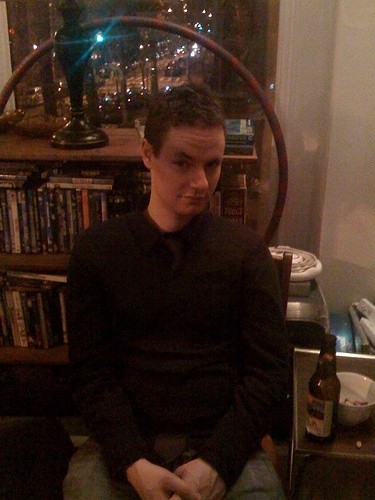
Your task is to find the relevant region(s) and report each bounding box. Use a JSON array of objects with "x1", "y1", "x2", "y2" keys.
[
  {"x1": 0, "y1": 268, "x2": 68, "y2": 350},
  {"x1": 135, "y1": 118, "x2": 258, "y2": 156},
  {"x1": 0, "y1": 162, "x2": 248, "y2": 255}
]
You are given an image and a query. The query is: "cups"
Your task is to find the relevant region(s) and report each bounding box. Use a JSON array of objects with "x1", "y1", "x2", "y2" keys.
[{"x1": 336, "y1": 372, "x2": 375, "y2": 426}]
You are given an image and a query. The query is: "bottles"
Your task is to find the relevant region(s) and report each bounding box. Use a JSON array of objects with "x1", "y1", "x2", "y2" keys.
[{"x1": 305, "y1": 334, "x2": 341, "y2": 444}]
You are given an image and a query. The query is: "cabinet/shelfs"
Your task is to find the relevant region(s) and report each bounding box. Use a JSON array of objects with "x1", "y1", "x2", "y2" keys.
[{"x1": 0, "y1": 128, "x2": 258, "y2": 365}]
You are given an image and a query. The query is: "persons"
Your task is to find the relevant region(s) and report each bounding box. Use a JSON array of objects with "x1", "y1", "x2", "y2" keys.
[{"x1": 64, "y1": 80, "x2": 290, "y2": 500}]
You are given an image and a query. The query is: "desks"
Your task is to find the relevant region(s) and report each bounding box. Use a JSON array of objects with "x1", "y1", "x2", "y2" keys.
[{"x1": 293, "y1": 349, "x2": 374, "y2": 500}]
